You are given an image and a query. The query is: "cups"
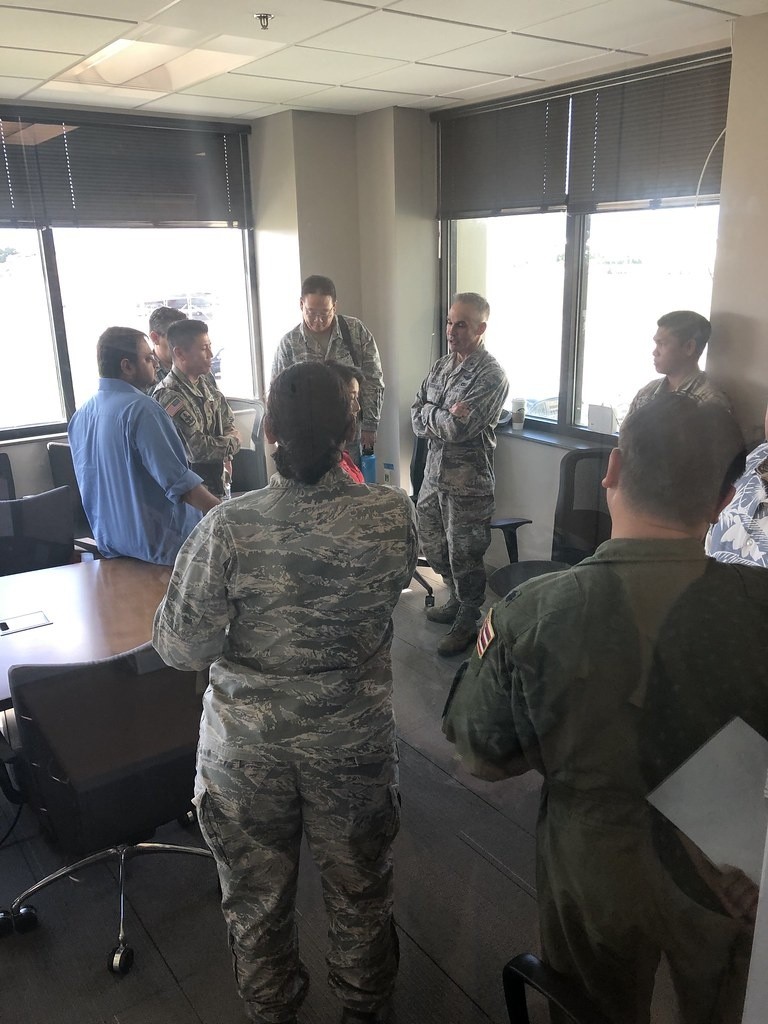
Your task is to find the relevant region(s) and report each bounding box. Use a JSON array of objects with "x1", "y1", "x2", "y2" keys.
[{"x1": 512, "y1": 398, "x2": 525, "y2": 430}]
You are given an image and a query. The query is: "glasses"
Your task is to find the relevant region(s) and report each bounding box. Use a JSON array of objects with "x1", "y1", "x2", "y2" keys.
[{"x1": 304, "y1": 303, "x2": 334, "y2": 320}]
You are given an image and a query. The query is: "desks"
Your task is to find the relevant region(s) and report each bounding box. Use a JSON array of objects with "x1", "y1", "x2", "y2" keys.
[{"x1": 0, "y1": 556, "x2": 175, "y2": 714}]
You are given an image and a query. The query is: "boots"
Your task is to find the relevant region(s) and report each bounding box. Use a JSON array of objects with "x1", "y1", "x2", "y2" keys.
[
  {"x1": 425, "y1": 594, "x2": 482, "y2": 624},
  {"x1": 439, "y1": 603, "x2": 478, "y2": 658}
]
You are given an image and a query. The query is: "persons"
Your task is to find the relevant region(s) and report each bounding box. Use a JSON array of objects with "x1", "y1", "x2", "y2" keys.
[
  {"x1": 152, "y1": 362, "x2": 419, "y2": 1024},
  {"x1": 411, "y1": 293, "x2": 509, "y2": 657},
  {"x1": 68, "y1": 327, "x2": 221, "y2": 566},
  {"x1": 271, "y1": 275, "x2": 386, "y2": 471},
  {"x1": 325, "y1": 360, "x2": 365, "y2": 486},
  {"x1": 441, "y1": 390, "x2": 768, "y2": 1023},
  {"x1": 715, "y1": 833, "x2": 766, "y2": 1024},
  {"x1": 152, "y1": 320, "x2": 241, "y2": 502},
  {"x1": 705, "y1": 395, "x2": 768, "y2": 570},
  {"x1": 144, "y1": 307, "x2": 189, "y2": 397},
  {"x1": 625, "y1": 310, "x2": 734, "y2": 417}
]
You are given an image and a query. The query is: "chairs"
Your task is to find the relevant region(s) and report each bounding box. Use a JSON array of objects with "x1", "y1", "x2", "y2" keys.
[
  {"x1": 1, "y1": 453, "x2": 74, "y2": 577},
  {"x1": 0, "y1": 641, "x2": 222, "y2": 975},
  {"x1": 407, "y1": 436, "x2": 435, "y2": 607},
  {"x1": 47, "y1": 442, "x2": 104, "y2": 564},
  {"x1": 226, "y1": 397, "x2": 268, "y2": 499},
  {"x1": 487, "y1": 447, "x2": 612, "y2": 602}
]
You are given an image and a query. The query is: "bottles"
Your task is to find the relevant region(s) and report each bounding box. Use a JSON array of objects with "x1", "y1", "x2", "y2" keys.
[{"x1": 361, "y1": 443, "x2": 376, "y2": 484}]
